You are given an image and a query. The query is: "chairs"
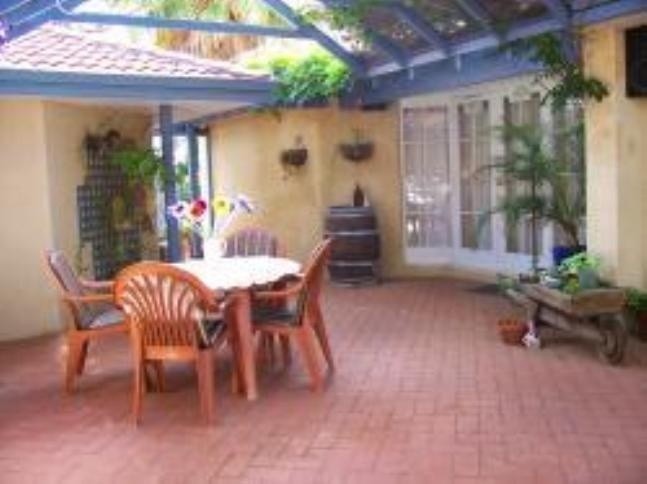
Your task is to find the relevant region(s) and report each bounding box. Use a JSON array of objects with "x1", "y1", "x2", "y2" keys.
[
  {"x1": 109, "y1": 258, "x2": 241, "y2": 432},
  {"x1": 41, "y1": 248, "x2": 158, "y2": 400},
  {"x1": 220, "y1": 226, "x2": 295, "y2": 370},
  {"x1": 244, "y1": 237, "x2": 338, "y2": 397}
]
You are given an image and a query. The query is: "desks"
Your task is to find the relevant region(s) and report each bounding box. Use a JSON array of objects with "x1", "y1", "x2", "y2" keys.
[{"x1": 162, "y1": 255, "x2": 303, "y2": 402}]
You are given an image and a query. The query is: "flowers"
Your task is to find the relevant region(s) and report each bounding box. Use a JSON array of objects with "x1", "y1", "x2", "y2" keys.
[{"x1": 166, "y1": 191, "x2": 259, "y2": 238}]
